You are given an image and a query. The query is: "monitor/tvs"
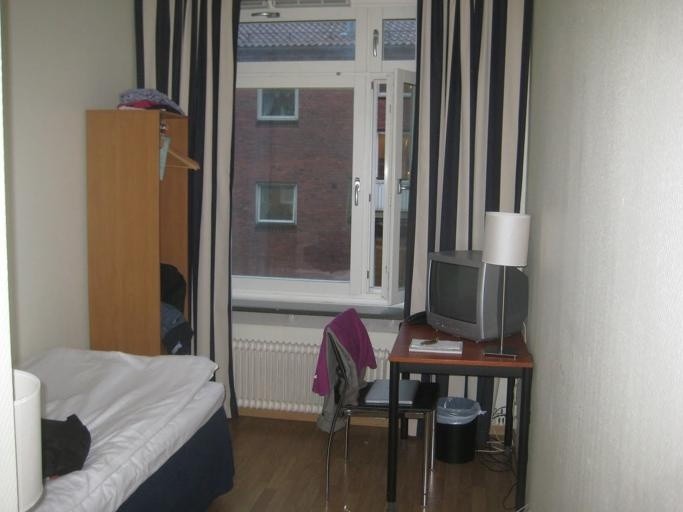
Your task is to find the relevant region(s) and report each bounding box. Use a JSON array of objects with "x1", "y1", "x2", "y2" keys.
[{"x1": 425, "y1": 249, "x2": 529, "y2": 344}]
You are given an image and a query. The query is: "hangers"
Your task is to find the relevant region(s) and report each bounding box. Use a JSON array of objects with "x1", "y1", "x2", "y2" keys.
[{"x1": 159, "y1": 130, "x2": 201, "y2": 171}]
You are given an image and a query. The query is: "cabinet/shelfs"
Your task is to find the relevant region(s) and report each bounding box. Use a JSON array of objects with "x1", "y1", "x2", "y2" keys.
[{"x1": 82, "y1": 106, "x2": 194, "y2": 355}]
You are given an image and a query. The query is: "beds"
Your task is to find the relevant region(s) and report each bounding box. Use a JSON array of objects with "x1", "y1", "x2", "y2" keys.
[{"x1": 16, "y1": 345, "x2": 240, "y2": 512}]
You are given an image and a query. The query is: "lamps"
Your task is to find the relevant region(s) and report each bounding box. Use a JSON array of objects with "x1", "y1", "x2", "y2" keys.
[{"x1": 486, "y1": 212, "x2": 534, "y2": 356}]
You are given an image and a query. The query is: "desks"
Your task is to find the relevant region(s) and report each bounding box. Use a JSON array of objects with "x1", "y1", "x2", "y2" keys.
[{"x1": 387, "y1": 320, "x2": 528, "y2": 511}]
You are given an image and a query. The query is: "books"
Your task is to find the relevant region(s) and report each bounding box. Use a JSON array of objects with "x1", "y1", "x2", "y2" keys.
[{"x1": 408, "y1": 337, "x2": 464, "y2": 356}]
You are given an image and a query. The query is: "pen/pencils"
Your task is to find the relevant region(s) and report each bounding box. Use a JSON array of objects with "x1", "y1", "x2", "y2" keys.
[{"x1": 421, "y1": 341, "x2": 436, "y2": 344}]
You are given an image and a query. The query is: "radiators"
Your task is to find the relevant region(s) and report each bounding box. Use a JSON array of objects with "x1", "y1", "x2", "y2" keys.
[{"x1": 229, "y1": 338, "x2": 388, "y2": 412}]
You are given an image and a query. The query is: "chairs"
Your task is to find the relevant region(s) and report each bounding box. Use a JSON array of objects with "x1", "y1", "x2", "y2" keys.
[{"x1": 321, "y1": 309, "x2": 440, "y2": 509}]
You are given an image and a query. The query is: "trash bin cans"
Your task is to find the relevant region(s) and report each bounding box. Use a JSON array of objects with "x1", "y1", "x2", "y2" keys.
[{"x1": 434, "y1": 397, "x2": 481, "y2": 464}]
